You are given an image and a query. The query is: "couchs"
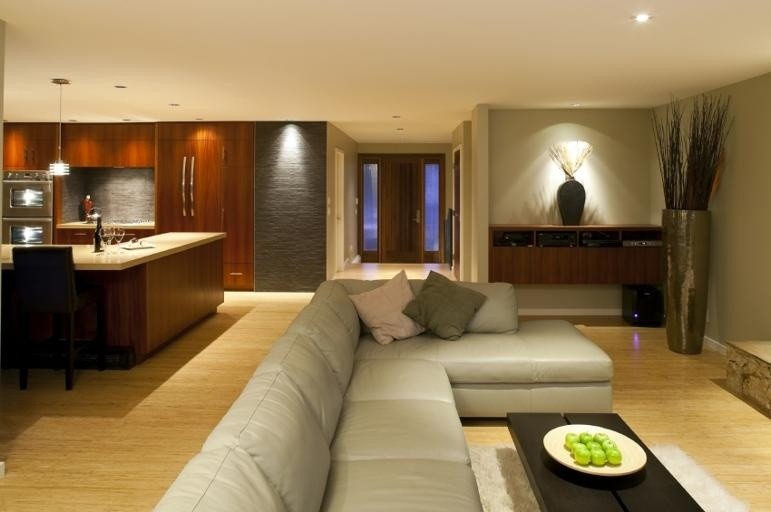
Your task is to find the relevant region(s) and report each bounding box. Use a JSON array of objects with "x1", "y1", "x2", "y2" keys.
[{"x1": 148, "y1": 278, "x2": 615, "y2": 512}]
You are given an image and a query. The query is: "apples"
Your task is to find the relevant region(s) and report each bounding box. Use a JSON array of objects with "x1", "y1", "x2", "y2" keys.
[{"x1": 565, "y1": 432, "x2": 621, "y2": 466}]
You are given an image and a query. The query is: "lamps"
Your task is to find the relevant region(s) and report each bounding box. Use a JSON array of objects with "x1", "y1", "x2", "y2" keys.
[{"x1": 49, "y1": 77, "x2": 70, "y2": 177}]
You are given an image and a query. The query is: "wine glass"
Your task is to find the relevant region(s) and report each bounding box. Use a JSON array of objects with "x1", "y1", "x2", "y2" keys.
[{"x1": 99, "y1": 224, "x2": 125, "y2": 256}]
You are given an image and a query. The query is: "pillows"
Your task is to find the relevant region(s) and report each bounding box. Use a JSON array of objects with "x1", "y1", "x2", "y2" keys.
[{"x1": 348, "y1": 270, "x2": 487, "y2": 345}]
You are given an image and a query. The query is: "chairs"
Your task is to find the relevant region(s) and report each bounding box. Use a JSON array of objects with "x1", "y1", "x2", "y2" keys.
[{"x1": 12, "y1": 247, "x2": 107, "y2": 390}]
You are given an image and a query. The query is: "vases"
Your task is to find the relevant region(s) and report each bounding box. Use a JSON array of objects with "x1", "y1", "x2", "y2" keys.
[
  {"x1": 557, "y1": 174, "x2": 585, "y2": 226},
  {"x1": 663, "y1": 209, "x2": 711, "y2": 355}
]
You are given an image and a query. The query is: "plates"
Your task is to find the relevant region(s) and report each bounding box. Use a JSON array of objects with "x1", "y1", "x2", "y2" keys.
[
  {"x1": 541, "y1": 423, "x2": 647, "y2": 477},
  {"x1": 121, "y1": 242, "x2": 155, "y2": 250}
]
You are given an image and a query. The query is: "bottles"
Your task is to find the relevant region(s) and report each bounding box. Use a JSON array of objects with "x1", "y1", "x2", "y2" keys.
[
  {"x1": 82, "y1": 195, "x2": 93, "y2": 219},
  {"x1": 92, "y1": 216, "x2": 105, "y2": 252}
]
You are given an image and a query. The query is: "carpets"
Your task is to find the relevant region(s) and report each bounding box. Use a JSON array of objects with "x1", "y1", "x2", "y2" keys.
[{"x1": 468, "y1": 436, "x2": 751, "y2": 512}]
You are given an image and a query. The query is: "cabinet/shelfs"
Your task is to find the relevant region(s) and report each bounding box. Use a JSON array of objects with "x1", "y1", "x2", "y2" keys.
[
  {"x1": 3, "y1": 122, "x2": 60, "y2": 171},
  {"x1": 223, "y1": 123, "x2": 254, "y2": 290},
  {"x1": 158, "y1": 124, "x2": 222, "y2": 234},
  {"x1": 489, "y1": 224, "x2": 664, "y2": 286},
  {"x1": 61, "y1": 124, "x2": 155, "y2": 168}
]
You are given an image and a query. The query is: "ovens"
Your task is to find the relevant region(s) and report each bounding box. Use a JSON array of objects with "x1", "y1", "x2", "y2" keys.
[{"x1": 2, "y1": 169, "x2": 53, "y2": 246}]
